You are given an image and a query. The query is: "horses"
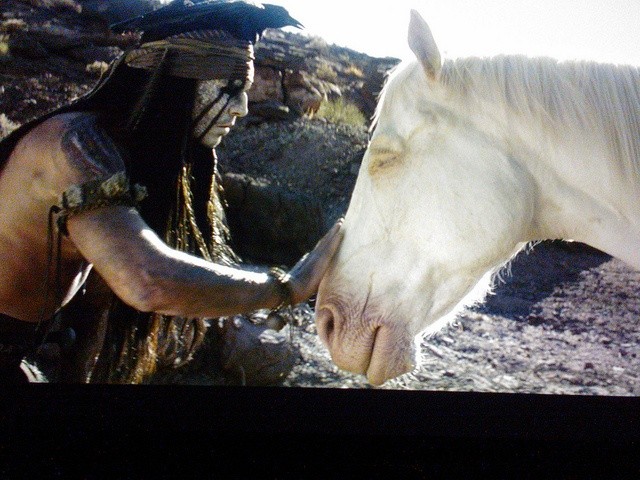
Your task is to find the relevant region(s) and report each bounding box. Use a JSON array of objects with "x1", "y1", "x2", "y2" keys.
[{"x1": 315, "y1": 7, "x2": 639, "y2": 386}]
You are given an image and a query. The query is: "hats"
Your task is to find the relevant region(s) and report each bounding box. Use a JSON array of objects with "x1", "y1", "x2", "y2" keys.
[{"x1": 110, "y1": 0, "x2": 302, "y2": 46}]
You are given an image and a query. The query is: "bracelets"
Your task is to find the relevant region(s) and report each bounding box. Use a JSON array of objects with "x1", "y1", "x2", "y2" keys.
[{"x1": 268, "y1": 267, "x2": 293, "y2": 313}]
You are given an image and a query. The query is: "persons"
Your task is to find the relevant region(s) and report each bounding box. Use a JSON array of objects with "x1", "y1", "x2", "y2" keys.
[{"x1": 0, "y1": 1, "x2": 345, "y2": 384}]
